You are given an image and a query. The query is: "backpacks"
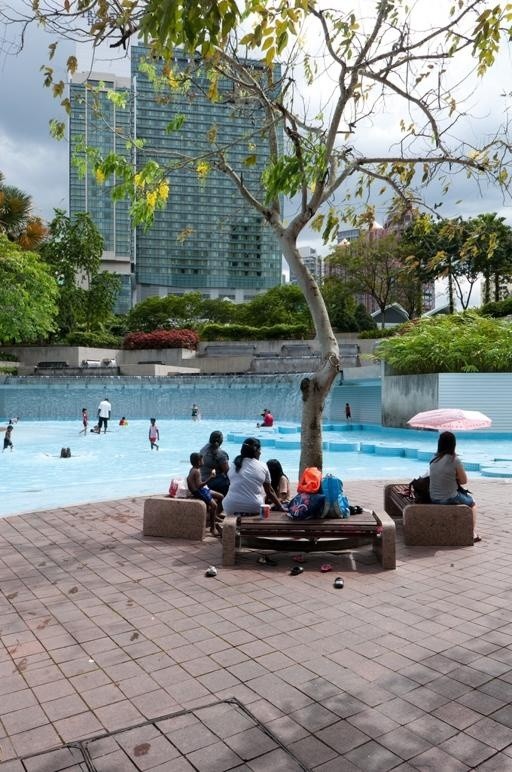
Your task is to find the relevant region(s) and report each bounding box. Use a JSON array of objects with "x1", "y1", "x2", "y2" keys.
[{"x1": 321, "y1": 472, "x2": 350, "y2": 519}]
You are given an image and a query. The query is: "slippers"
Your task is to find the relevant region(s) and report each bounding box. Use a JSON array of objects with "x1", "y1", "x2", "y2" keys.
[{"x1": 474, "y1": 534, "x2": 481, "y2": 542}]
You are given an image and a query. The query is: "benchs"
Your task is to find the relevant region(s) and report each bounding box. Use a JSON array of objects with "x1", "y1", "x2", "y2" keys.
[
  {"x1": 382, "y1": 479, "x2": 474, "y2": 549},
  {"x1": 142, "y1": 487, "x2": 208, "y2": 542},
  {"x1": 222, "y1": 508, "x2": 394, "y2": 570}
]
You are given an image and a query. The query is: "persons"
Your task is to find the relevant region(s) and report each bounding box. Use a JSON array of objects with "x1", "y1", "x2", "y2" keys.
[
  {"x1": 148, "y1": 418, "x2": 160, "y2": 451},
  {"x1": 187, "y1": 453, "x2": 222, "y2": 537},
  {"x1": 1, "y1": 416, "x2": 19, "y2": 453},
  {"x1": 345, "y1": 402, "x2": 352, "y2": 425},
  {"x1": 79, "y1": 397, "x2": 127, "y2": 436},
  {"x1": 221, "y1": 437, "x2": 289, "y2": 518},
  {"x1": 263, "y1": 458, "x2": 291, "y2": 511},
  {"x1": 191, "y1": 404, "x2": 197, "y2": 423},
  {"x1": 257, "y1": 409, "x2": 273, "y2": 428},
  {"x1": 200, "y1": 430, "x2": 230, "y2": 518},
  {"x1": 428, "y1": 430, "x2": 482, "y2": 542}
]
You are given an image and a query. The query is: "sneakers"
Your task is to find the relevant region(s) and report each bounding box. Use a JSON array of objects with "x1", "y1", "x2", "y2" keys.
[
  {"x1": 350, "y1": 505, "x2": 364, "y2": 513},
  {"x1": 335, "y1": 577, "x2": 344, "y2": 588},
  {"x1": 292, "y1": 555, "x2": 304, "y2": 563},
  {"x1": 320, "y1": 564, "x2": 331, "y2": 570},
  {"x1": 206, "y1": 565, "x2": 217, "y2": 575},
  {"x1": 258, "y1": 557, "x2": 276, "y2": 565},
  {"x1": 291, "y1": 566, "x2": 304, "y2": 573}
]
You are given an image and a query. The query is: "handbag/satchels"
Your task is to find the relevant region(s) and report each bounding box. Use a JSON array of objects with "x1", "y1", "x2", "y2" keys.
[
  {"x1": 457, "y1": 479, "x2": 469, "y2": 494},
  {"x1": 408, "y1": 478, "x2": 431, "y2": 505},
  {"x1": 288, "y1": 466, "x2": 323, "y2": 520}
]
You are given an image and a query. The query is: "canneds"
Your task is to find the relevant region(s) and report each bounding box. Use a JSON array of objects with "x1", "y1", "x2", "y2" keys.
[{"x1": 260, "y1": 505, "x2": 270, "y2": 520}]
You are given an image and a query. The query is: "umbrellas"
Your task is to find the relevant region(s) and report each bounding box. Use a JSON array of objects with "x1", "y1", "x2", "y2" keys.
[{"x1": 407, "y1": 406, "x2": 492, "y2": 434}]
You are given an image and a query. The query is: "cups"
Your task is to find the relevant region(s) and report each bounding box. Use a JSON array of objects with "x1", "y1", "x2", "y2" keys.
[{"x1": 259, "y1": 504, "x2": 270, "y2": 519}]
